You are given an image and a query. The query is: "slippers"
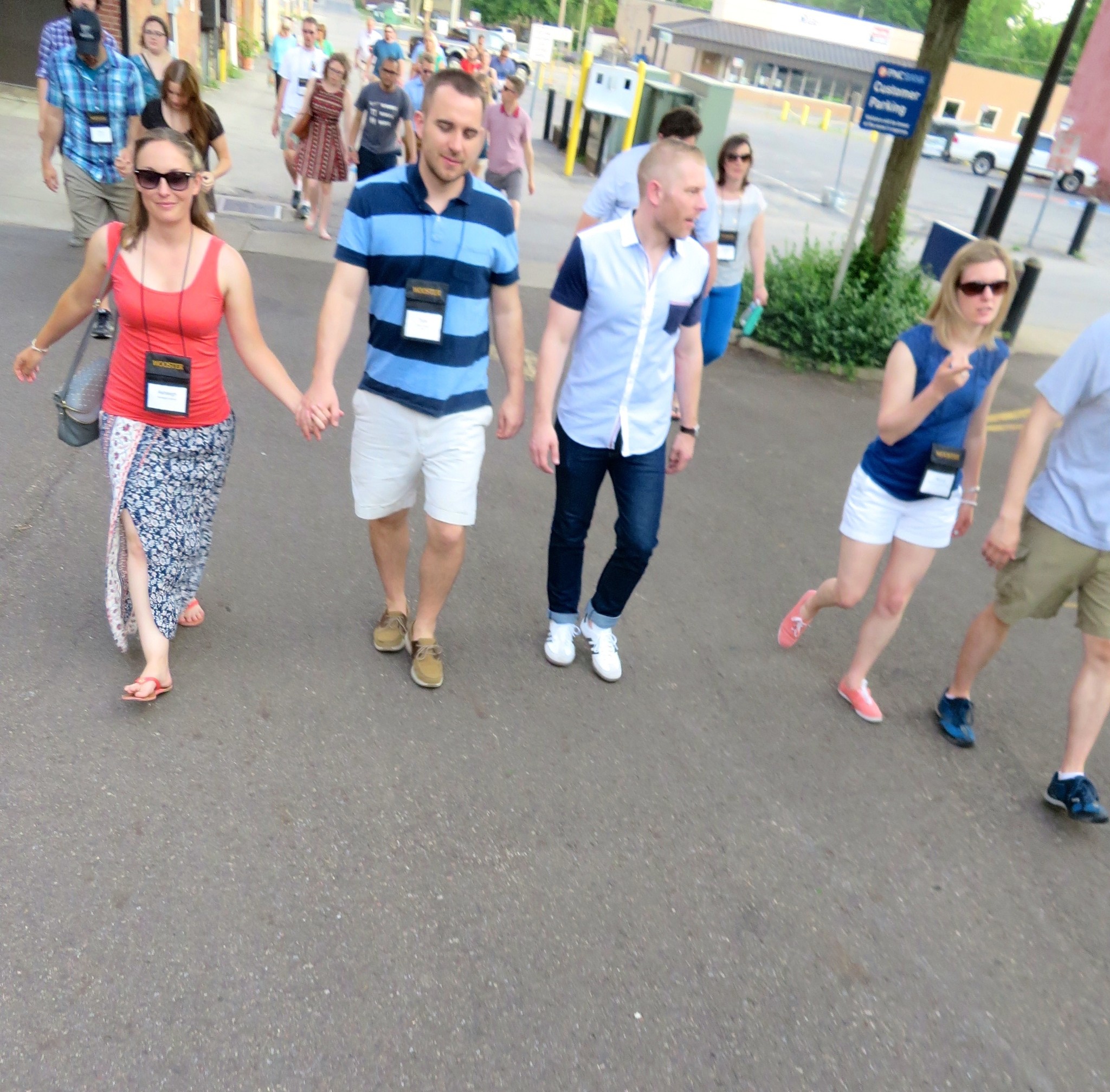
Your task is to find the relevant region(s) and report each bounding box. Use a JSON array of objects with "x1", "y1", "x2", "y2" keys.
[
  {"x1": 179, "y1": 600, "x2": 205, "y2": 626},
  {"x1": 306, "y1": 213, "x2": 316, "y2": 231},
  {"x1": 319, "y1": 230, "x2": 332, "y2": 240},
  {"x1": 122, "y1": 677, "x2": 173, "y2": 701}
]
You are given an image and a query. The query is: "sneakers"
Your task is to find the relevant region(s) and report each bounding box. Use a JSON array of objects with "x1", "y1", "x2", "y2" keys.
[
  {"x1": 837, "y1": 674, "x2": 884, "y2": 723},
  {"x1": 934, "y1": 686, "x2": 976, "y2": 749},
  {"x1": 373, "y1": 600, "x2": 410, "y2": 651},
  {"x1": 404, "y1": 619, "x2": 444, "y2": 687},
  {"x1": 88, "y1": 308, "x2": 115, "y2": 339},
  {"x1": 778, "y1": 590, "x2": 817, "y2": 648},
  {"x1": 580, "y1": 616, "x2": 622, "y2": 682},
  {"x1": 1042, "y1": 769, "x2": 1109, "y2": 824},
  {"x1": 544, "y1": 619, "x2": 576, "y2": 666}
]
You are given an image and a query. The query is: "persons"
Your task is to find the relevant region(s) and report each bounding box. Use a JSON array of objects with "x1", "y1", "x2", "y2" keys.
[
  {"x1": 130, "y1": 14, "x2": 180, "y2": 102},
  {"x1": 935, "y1": 309, "x2": 1110, "y2": 823},
  {"x1": 302, "y1": 67, "x2": 529, "y2": 690},
  {"x1": 573, "y1": 106, "x2": 720, "y2": 299},
  {"x1": 42, "y1": 9, "x2": 151, "y2": 340},
  {"x1": 267, "y1": 11, "x2": 536, "y2": 239},
  {"x1": 36, "y1": 0, "x2": 122, "y2": 247},
  {"x1": 529, "y1": 135, "x2": 709, "y2": 682},
  {"x1": 114, "y1": 59, "x2": 234, "y2": 226},
  {"x1": 10, "y1": 128, "x2": 344, "y2": 700},
  {"x1": 672, "y1": 133, "x2": 769, "y2": 419},
  {"x1": 779, "y1": 237, "x2": 1011, "y2": 721}
]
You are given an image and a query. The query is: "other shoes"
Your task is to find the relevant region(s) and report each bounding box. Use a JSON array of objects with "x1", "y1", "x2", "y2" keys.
[
  {"x1": 298, "y1": 199, "x2": 310, "y2": 219},
  {"x1": 291, "y1": 184, "x2": 301, "y2": 209},
  {"x1": 68, "y1": 232, "x2": 85, "y2": 247},
  {"x1": 671, "y1": 392, "x2": 681, "y2": 421}
]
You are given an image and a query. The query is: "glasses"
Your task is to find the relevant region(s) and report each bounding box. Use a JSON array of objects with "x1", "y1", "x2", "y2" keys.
[
  {"x1": 134, "y1": 169, "x2": 194, "y2": 191},
  {"x1": 726, "y1": 152, "x2": 751, "y2": 162},
  {"x1": 142, "y1": 29, "x2": 166, "y2": 37},
  {"x1": 303, "y1": 29, "x2": 316, "y2": 34},
  {"x1": 956, "y1": 277, "x2": 1009, "y2": 297},
  {"x1": 328, "y1": 66, "x2": 344, "y2": 76},
  {"x1": 381, "y1": 67, "x2": 398, "y2": 76},
  {"x1": 422, "y1": 67, "x2": 434, "y2": 74},
  {"x1": 504, "y1": 85, "x2": 515, "y2": 94}
]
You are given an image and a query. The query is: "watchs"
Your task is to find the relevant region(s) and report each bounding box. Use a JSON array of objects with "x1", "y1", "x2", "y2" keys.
[{"x1": 679, "y1": 423, "x2": 701, "y2": 438}]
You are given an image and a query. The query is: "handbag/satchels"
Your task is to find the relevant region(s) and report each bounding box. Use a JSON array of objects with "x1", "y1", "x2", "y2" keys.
[
  {"x1": 292, "y1": 113, "x2": 314, "y2": 139},
  {"x1": 53, "y1": 356, "x2": 110, "y2": 447}
]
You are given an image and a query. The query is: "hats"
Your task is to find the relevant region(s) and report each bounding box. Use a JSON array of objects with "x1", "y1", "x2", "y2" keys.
[{"x1": 71, "y1": 8, "x2": 101, "y2": 57}]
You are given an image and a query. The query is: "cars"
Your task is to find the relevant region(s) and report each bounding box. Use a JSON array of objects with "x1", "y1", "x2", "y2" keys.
[
  {"x1": 409, "y1": 22, "x2": 531, "y2": 85},
  {"x1": 921, "y1": 121, "x2": 963, "y2": 164}
]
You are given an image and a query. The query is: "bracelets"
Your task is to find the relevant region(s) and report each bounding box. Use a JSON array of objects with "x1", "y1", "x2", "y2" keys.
[
  {"x1": 959, "y1": 486, "x2": 981, "y2": 495},
  {"x1": 31, "y1": 339, "x2": 51, "y2": 354},
  {"x1": 960, "y1": 499, "x2": 978, "y2": 507}
]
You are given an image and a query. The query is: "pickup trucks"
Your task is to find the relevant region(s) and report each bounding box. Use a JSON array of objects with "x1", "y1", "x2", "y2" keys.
[{"x1": 948, "y1": 128, "x2": 1101, "y2": 195}]
